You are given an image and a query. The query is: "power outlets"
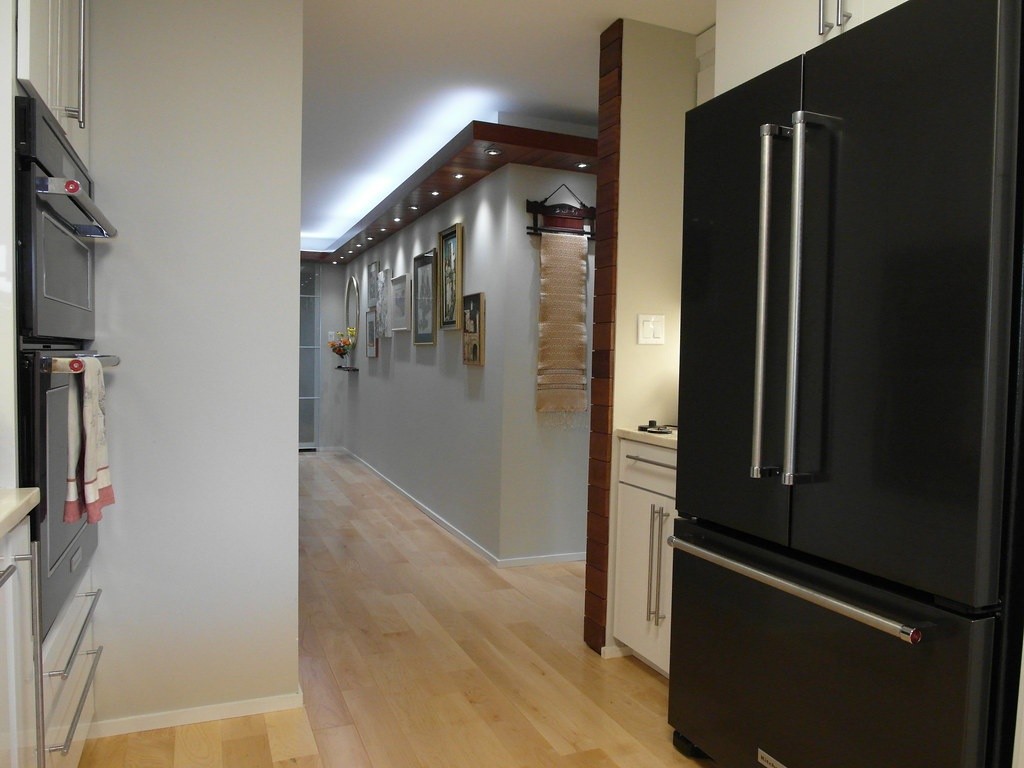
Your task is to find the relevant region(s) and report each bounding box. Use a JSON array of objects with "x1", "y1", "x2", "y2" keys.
[{"x1": 328, "y1": 332, "x2": 335, "y2": 341}]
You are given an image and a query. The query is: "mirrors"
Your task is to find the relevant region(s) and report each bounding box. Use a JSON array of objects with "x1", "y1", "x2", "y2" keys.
[{"x1": 345, "y1": 276, "x2": 360, "y2": 349}]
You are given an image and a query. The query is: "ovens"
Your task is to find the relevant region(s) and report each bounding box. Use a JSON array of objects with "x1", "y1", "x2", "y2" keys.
[{"x1": 14, "y1": 97, "x2": 121, "y2": 640}]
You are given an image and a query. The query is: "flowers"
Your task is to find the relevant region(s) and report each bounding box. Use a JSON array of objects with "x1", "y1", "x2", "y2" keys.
[
  {"x1": 327, "y1": 331, "x2": 351, "y2": 358},
  {"x1": 348, "y1": 327, "x2": 356, "y2": 337}
]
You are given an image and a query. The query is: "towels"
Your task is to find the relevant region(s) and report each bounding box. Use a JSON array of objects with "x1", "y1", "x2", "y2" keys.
[{"x1": 63, "y1": 356, "x2": 115, "y2": 526}]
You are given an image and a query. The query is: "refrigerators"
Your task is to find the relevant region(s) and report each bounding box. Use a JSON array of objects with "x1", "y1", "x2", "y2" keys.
[{"x1": 666, "y1": 2, "x2": 1024, "y2": 767}]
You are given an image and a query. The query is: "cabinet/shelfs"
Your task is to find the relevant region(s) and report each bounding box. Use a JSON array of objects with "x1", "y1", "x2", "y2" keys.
[
  {"x1": 40, "y1": 565, "x2": 104, "y2": 768},
  {"x1": 613, "y1": 438, "x2": 677, "y2": 681},
  {"x1": 714, "y1": 0, "x2": 909, "y2": 98},
  {"x1": 0, "y1": 0, "x2": 91, "y2": 170}
]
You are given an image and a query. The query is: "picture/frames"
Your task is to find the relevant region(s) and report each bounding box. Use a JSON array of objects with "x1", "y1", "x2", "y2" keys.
[
  {"x1": 390, "y1": 273, "x2": 411, "y2": 332},
  {"x1": 436, "y1": 223, "x2": 462, "y2": 331},
  {"x1": 367, "y1": 260, "x2": 380, "y2": 308},
  {"x1": 412, "y1": 247, "x2": 436, "y2": 345},
  {"x1": 366, "y1": 310, "x2": 378, "y2": 358},
  {"x1": 460, "y1": 292, "x2": 485, "y2": 367}
]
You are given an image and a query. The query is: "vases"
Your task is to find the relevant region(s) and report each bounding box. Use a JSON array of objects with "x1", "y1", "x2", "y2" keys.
[{"x1": 336, "y1": 355, "x2": 347, "y2": 366}]
